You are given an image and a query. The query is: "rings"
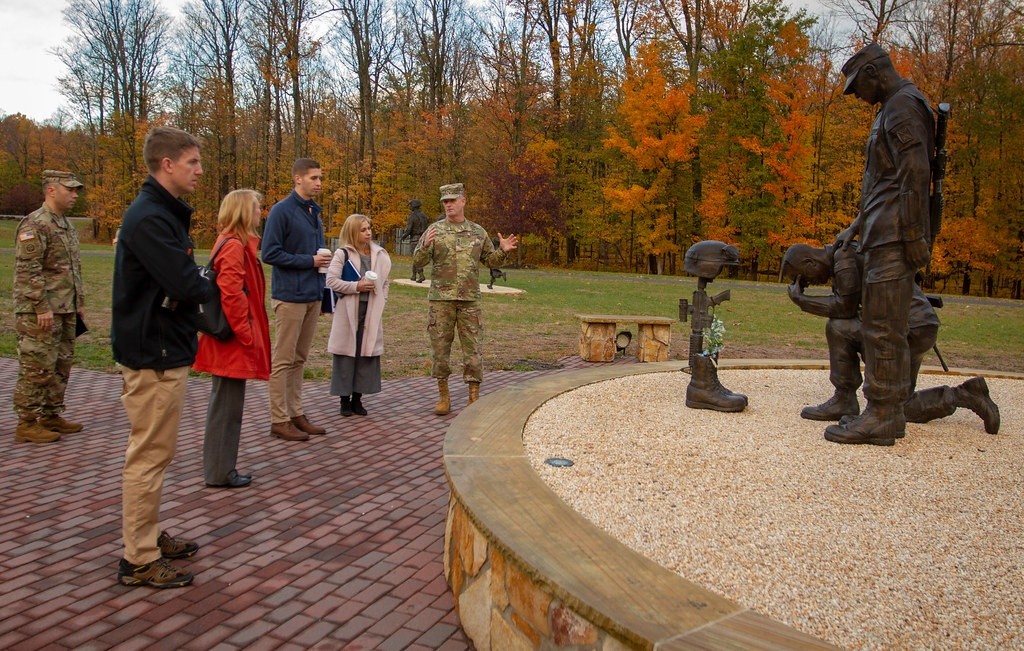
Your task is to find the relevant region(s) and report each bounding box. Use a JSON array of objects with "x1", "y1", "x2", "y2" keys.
[{"x1": 325, "y1": 261, "x2": 327, "y2": 265}]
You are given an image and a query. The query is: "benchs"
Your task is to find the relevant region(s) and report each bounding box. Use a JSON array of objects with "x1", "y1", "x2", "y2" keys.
[{"x1": 575, "y1": 313, "x2": 676, "y2": 362}]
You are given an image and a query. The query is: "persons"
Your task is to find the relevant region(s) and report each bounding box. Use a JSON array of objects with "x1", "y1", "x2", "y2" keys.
[
  {"x1": 111, "y1": 126, "x2": 213, "y2": 588},
  {"x1": 487, "y1": 237, "x2": 506, "y2": 289},
  {"x1": 12, "y1": 170, "x2": 85, "y2": 443},
  {"x1": 326, "y1": 214, "x2": 392, "y2": 417},
  {"x1": 190, "y1": 189, "x2": 272, "y2": 488},
  {"x1": 781, "y1": 241, "x2": 1001, "y2": 434},
  {"x1": 261, "y1": 159, "x2": 332, "y2": 440},
  {"x1": 824, "y1": 42, "x2": 936, "y2": 445},
  {"x1": 402, "y1": 199, "x2": 428, "y2": 283},
  {"x1": 413, "y1": 184, "x2": 518, "y2": 414}
]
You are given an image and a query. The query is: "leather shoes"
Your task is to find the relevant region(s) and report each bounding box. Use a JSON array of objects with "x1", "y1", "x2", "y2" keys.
[
  {"x1": 206, "y1": 477, "x2": 252, "y2": 488},
  {"x1": 290, "y1": 415, "x2": 326, "y2": 434},
  {"x1": 271, "y1": 421, "x2": 309, "y2": 441},
  {"x1": 237, "y1": 474, "x2": 252, "y2": 479}
]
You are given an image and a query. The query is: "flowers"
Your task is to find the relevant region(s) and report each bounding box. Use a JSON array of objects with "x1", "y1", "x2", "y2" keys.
[{"x1": 702, "y1": 313, "x2": 726, "y2": 357}]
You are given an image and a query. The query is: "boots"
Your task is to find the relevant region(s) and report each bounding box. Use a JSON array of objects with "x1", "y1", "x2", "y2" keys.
[
  {"x1": 351, "y1": 392, "x2": 367, "y2": 415},
  {"x1": 467, "y1": 382, "x2": 479, "y2": 406},
  {"x1": 340, "y1": 395, "x2": 352, "y2": 416},
  {"x1": 434, "y1": 379, "x2": 451, "y2": 415}
]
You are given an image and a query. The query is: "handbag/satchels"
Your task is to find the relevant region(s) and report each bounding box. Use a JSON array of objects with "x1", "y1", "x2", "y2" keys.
[{"x1": 197, "y1": 236, "x2": 248, "y2": 342}]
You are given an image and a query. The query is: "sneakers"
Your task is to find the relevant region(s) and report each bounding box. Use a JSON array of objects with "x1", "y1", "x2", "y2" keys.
[
  {"x1": 15, "y1": 417, "x2": 61, "y2": 444},
  {"x1": 39, "y1": 414, "x2": 83, "y2": 433},
  {"x1": 156, "y1": 531, "x2": 200, "y2": 558},
  {"x1": 118, "y1": 557, "x2": 194, "y2": 588}
]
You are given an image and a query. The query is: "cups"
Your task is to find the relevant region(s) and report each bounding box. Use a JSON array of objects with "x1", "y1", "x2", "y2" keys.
[
  {"x1": 317, "y1": 248, "x2": 332, "y2": 274},
  {"x1": 365, "y1": 270, "x2": 377, "y2": 282}
]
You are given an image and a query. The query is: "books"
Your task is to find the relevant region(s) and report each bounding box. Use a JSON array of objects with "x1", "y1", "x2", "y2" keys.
[
  {"x1": 334, "y1": 259, "x2": 362, "y2": 299},
  {"x1": 321, "y1": 287, "x2": 335, "y2": 314}
]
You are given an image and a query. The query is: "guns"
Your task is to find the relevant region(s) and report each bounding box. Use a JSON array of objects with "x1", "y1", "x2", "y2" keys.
[
  {"x1": 925, "y1": 102, "x2": 951, "y2": 277},
  {"x1": 926, "y1": 294, "x2": 950, "y2": 373},
  {"x1": 678, "y1": 277, "x2": 732, "y2": 375}
]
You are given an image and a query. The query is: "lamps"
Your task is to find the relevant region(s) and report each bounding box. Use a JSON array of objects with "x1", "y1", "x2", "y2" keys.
[{"x1": 616, "y1": 331, "x2": 632, "y2": 351}]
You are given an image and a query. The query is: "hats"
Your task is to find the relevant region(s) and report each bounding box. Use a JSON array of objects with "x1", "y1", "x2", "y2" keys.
[
  {"x1": 42, "y1": 170, "x2": 83, "y2": 190},
  {"x1": 439, "y1": 183, "x2": 465, "y2": 201}
]
